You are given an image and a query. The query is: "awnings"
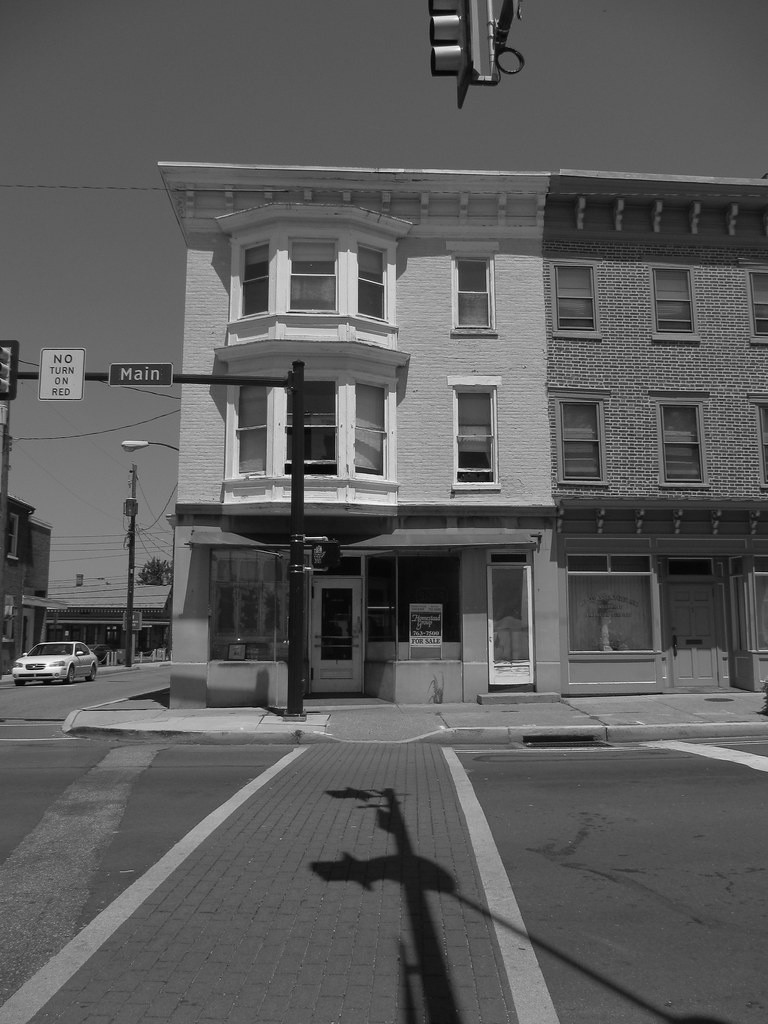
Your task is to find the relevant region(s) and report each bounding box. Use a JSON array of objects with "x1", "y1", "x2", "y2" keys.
[
  {"x1": 22, "y1": 595, "x2": 68, "y2": 610},
  {"x1": 189, "y1": 530, "x2": 537, "y2": 552}
]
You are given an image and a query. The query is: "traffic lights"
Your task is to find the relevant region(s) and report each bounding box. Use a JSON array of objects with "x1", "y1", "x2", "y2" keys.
[
  {"x1": 0, "y1": 339, "x2": 19, "y2": 399},
  {"x1": 427, "y1": 1, "x2": 474, "y2": 109},
  {"x1": 311, "y1": 540, "x2": 341, "y2": 569}
]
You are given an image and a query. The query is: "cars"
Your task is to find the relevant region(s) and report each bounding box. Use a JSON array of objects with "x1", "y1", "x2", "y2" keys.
[
  {"x1": 86, "y1": 645, "x2": 113, "y2": 664},
  {"x1": 12, "y1": 641, "x2": 99, "y2": 687}
]
